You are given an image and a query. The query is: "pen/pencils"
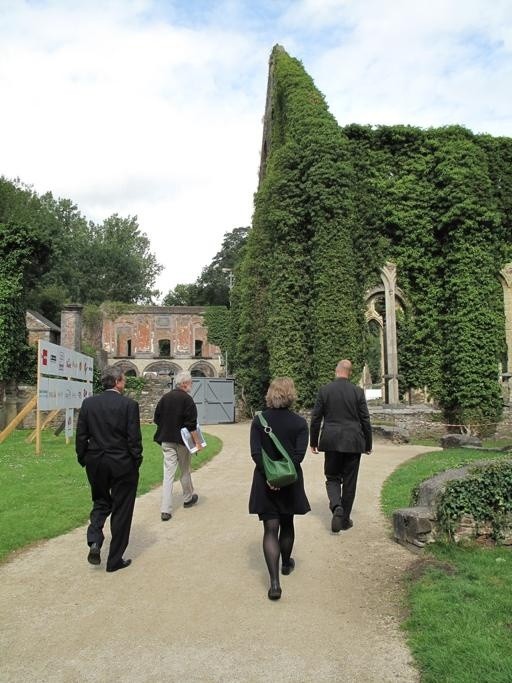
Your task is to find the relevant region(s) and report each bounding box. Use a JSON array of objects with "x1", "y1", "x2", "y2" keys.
[{"x1": 196, "y1": 451, "x2": 197, "y2": 456}]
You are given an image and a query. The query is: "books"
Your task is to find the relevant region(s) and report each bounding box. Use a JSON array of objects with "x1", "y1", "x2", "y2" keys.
[{"x1": 181, "y1": 424, "x2": 208, "y2": 454}]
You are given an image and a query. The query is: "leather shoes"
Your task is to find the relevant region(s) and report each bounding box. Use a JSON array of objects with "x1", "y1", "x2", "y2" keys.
[
  {"x1": 331, "y1": 505, "x2": 345, "y2": 532},
  {"x1": 161, "y1": 512, "x2": 171, "y2": 521},
  {"x1": 267, "y1": 586, "x2": 282, "y2": 600},
  {"x1": 343, "y1": 518, "x2": 353, "y2": 530},
  {"x1": 183, "y1": 494, "x2": 198, "y2": 508},
  {"x1": 87, "y1": 541, "x2": 101, "y2": 565},
  {"x1": 106, "y1": 558, "x2": 132, "y2": 572},
  {"x1": 281, "y1": 558, "x2": 295, "y2": 575}
]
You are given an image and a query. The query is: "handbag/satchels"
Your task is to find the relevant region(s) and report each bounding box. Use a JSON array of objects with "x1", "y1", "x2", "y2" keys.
[{"x1": 257, "y1": 411, "x2": 299, "y2": 488}]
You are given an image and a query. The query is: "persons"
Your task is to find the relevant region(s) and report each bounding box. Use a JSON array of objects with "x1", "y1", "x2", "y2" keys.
[
  {"x1": 152, "y1": 370, "x2": 205, "y2": 521},
  {"x1": 247, "y1": 375, "x2": 312, "y2": 600},
  {"x1": 74, "y1": 363, "x2": 144, "y2": 574},
  {"x1": 309, "y1": 358, "x2": 374, "y2": 533}
]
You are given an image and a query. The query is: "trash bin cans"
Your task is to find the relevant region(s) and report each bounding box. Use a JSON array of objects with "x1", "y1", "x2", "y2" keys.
[{"x1": 7, "y1": 399, "x2": 33, "y2": 430}]
